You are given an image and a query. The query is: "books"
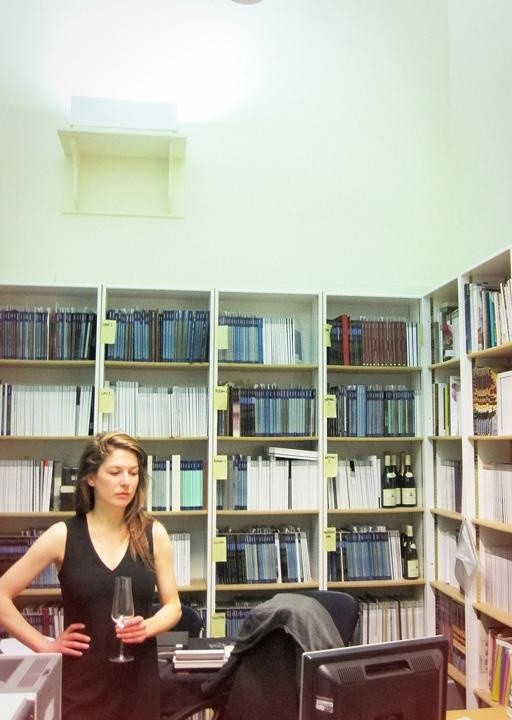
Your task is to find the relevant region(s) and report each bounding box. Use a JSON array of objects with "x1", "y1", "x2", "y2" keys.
[
  {"x1": 167, "y1": 530, "x2": 208, "y2": 639},
  {"x1": 218, "y1": 381, "x2": 317, "y2": 437},
  {"x1": 1, "y1": 304, "x2": 97, "y2": 361},
  {"x1": 326, "y1": 381, "x2": 416, "y2": 438},
  {"x1": 0, "y1": 458, "x2": 81, "y2": 512},
  {"x1": 327, "y1": 523, "x2": 404, "y2": 583},
  {"x1": 218, "y1": 311, "x2": 306, "y2": 365},
  {"x1": 352, "y1": 594, "x2": 425, "y2": 646},
  {"x1": 102, "y1": 379, "x2": 208, "y2": 436},
  {"x1": 216, "y1": 524, "x2": 311, "y2": 584},
  {"x1": 141, "y1": 453, "x2": 204, "y2": 512},
  {"x1": 214, "y1": 596, "x2": 273, "y2": 639},
  {"x1": 106, "y1": 309, "x2": 210, "y2": 364},
  {"x1": 1, "y1": 382, "x2": 93, "y2": 436},
  {"x1": 431, "y1": 279, "x2": 511, "y2": 712},
  {"x1": 0, "y1": 528, "x2": 60, "y2": 587},
  {"x1": 20, "y1": 601, "x2": 65, "y2": 639},
  {"x1": 325, "y1": 313, "x2": 420, "y2": 367},
  {"x1": 328, "y1": 454, "x2": 383, "y2": 509},
  {"x1": 216, "y1": 446, "x2": 319, "y2": 511}
]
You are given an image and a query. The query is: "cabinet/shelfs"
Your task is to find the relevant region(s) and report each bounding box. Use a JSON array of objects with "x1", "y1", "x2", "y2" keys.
[
  {"x1": 0, "y1": 282, "x2": 103, "y2": 642},
  {"x1": 323, "y1": 294, "x2": 426, "y2": 647},
  {"x1": 460, "y1": 245, "x2": 510, "y2": 712},
  {"x1": 212, "y1": 290, "x2": 323, "y2": 637},
  {"x1": 422, "y1": 275, "x2": 463, "y2": 710},
  {"x1": 97, "y1": 285, "x2": 213, "y2": 639}
]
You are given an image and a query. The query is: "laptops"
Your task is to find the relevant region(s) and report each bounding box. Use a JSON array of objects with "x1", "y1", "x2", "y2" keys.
[{"x1": 155, "y1": 631, "x2": 189, "y2": 650}]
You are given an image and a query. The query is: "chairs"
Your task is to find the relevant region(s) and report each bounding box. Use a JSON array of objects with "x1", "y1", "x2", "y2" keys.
[
  {"x1": 163, "y1": 607, "x2": 303, "y2": 720},
  {"x1": 259, "y1": 589, "x2": 361, "y2": 647},
  {"x1": 149, "y1": 604, "x2": 203, "y2": 639}
]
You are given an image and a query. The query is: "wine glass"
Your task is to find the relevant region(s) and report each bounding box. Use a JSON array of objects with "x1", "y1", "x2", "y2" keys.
[{"x1": 108, "y1": 576, "x2": 136, "y2": 664}]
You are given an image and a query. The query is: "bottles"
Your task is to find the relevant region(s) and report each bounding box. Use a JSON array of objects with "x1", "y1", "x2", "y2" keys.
[
  {"x1": 404, "y1": 525, "x2": 419, "y2": 579},
  {"x1": 401, "y1": 454, "x2": 416, "y2": 507},
  {"x1": 391, "y1": 453, "x2": 402, "y2": 506},
  {"x1": 400, "y1": 524, "x2": 407, "y2": 577},
  {"x1": 381, "y1": 451, "x2": 396, "y2": 507},
  {"x1": 399, "y1": 451, "x2": 405, "y2": 477}
]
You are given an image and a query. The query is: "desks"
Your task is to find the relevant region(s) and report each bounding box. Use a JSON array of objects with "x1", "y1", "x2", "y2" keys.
[{"x1": 157, "y1": 637, "x2": 237, "y2": 720}]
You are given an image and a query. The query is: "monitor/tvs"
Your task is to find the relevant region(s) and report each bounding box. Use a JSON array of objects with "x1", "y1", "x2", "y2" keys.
[{"x1": 299, "y1": 635, "x2": 449, "y2": 720}]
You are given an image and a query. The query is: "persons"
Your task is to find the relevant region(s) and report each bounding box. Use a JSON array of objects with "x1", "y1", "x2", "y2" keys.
[{"x1": 0, "y1": 432, "x2": 183, "y2": 720}]
